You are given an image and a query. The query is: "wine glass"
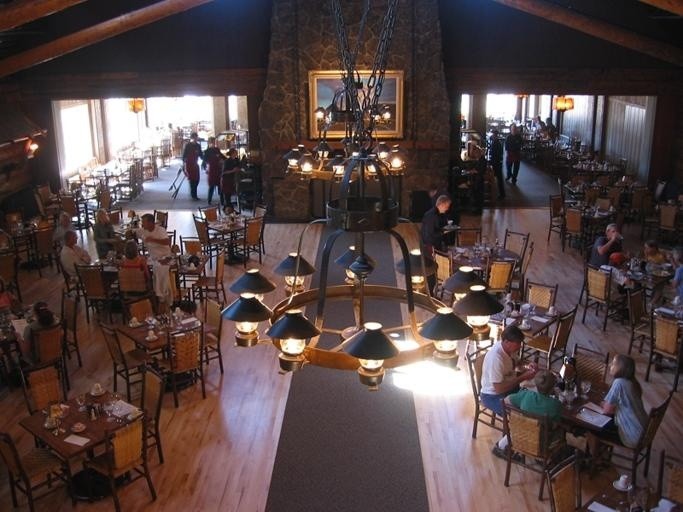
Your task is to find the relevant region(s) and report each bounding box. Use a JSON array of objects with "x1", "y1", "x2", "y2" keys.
[
  {"x1": 75, "y1": 391, "x2": 88, "y2": 413},
  {"x1": 110, "y1": 392, "x2": 124, "y2": 424},
  {"x1": 104, "y1": 401, "x2": 115, "y2": 423},
  {"x1": 564, "y1": 389, "x2": 576, "y2": 411}
]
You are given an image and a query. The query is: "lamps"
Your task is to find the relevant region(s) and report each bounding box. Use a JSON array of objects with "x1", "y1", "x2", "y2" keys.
[
  {"x1": 221, "y1": 0, "x2": 504, "y2": 393},
  {"x1": 281, "y1": 0, "x2": 406, "y2": 183},
  {"x1": 552, "y1": 94, "x2": 574, "y2": 113},
  {"x1": 128, "y1": 98, "x2": 144, "y2": 113}
]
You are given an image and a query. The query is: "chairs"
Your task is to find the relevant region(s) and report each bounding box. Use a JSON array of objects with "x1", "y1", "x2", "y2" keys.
[
  {"x1": 505, "y1": 228, "x2": 530, "y2": 261},
  {"x1": 141, "y1": 363, "x2": 166, "y2": 464},
  {"x1": 83, "y1": 409, "x2": 156, "y2": 512},
  {"x1": 509, "y1": 241, "x2": 534, "y2": 301},
  {"x1": 193, "y1": 214, "x2": 223, "y2": 269},
  {"x1": 639, "y1": 192, "x2": 660, "y2": 241},
  {"x1": 548, "y1": 194, "x2": 566, "y2": 241},
  {"x1": 167, "y1": 230, "x2": 176, "y2": 248},
  {"x1": 37, "y1": 183, "x2": 52, "y2": 204},
  {"x1": 466, "y1": 338, "x2": 510, "y2": 438},
  {"x1": 29, "y1": 321, "x2": 65, "y2": 386},
  {"x1": 154, "y1": 210, "x2": 168, "y2": 228},
  {"x1": 523, "y1": 278, "x2": 559, "y2": 309},
  {"x1": 616, "y1": 213, "x2": 623, "y2": 245},
  {"x1": 254, "y1": 204, "x2": 268, "y2": 255},
  {"x1": 203, "y1": 296, "x2": 224, "y2": 375},
  {"x1": 232, "y1": 215, "x2": 264, "y2": 268},
  {"x1": 656, "y1": 449, "x2": 683, "y2": 504},
  {"x1": 645, "y1": 308, "x2": 683, "y2": 392},
  {"x1": 116, "y1": 263, "x2": 155, "y2": 325},
  {"x1": 31, "y1": 224, "x2": 60, "y2": 277},
  {"x1": 600, "y1": 391, "x2": 673, "y2": 485},
  {"x1": 192, "y1": 249, "x2": 227, "y2": 310},
  {"x1": 19, "y1": 354, "x2": 69, "y2": 448},
  {"x1": 0, "y1": 430, "x2": 63, "y2": 512},
  {"x1": 3, "y1": 207, "x2": 24, "y2": 225},
  {"x1": 627, "y1": 185, "x2": 648, "y2": 227},
  {"x1": 98, "y1": 321, "x2": 156, "y2": 402},
  {"x1": 521, "y1": 304, "x2": 578, "y2": 370},
  {"x1": 500, "y1": 399, "x2": 568, "y2": 501},
  {"x1": 32, "y1": 188, "x2": 48, "y2": 219},
  {"x1": 457, "y1": 226, "x2": 482, "y2": 247},
  {"x1": 627, "y1": 283, "x2": 655, "y2": 355},
  {"x1": 60, "y1": 289, "x2": 83, "y2": 367},
  {"x1": 574, "y1": 344, "x2": 610, "y2": 382},
  {"x1": 582, "y1": 259, "x2": 627, "y2": 331},
  {"x1": 595, "y1": 196, "x2": 614, "y2": 210},
  {"x1": 199, "y1": 203, "x2": 222, "y2": 220},
  {"x1": 0, "y1": 254, "x2": 23, "y2": 303},
  {"x1": 156, "y1": 322, "x2": 206, "y2": 408},
  {"x1": 658, "y1": 201, "x2": 680, "y2": 246},
  {"x1": 59, "y1": 119, "x2": 198, "y2": 210},
  {"x1": 122, "y1": 292, "x2": 158, "y2": 324},
  {"x1": 58, "y1": 252, "x2": 84, "y2": 302},
  {"x1": 579, "y1": 244, "x2": 593, "y2": 304},
  {"x1": 179, "y1": 235, "x2": 207, "y2": 289},
  {"x1": 74, "y1": 261, "x2": 121, "y2": 324},
  {"x1": 562, "y1": 207, "x2": 588, "y2": 255},
  {"x1": 544, "y1": 449, "x2": 581, "y2": 512},
  {"x1": 485, "y1": 257, "x2": 517, "y2": 295},
  {"x1": 432, "y1": 246, "x2": 453, "y2": 299}
]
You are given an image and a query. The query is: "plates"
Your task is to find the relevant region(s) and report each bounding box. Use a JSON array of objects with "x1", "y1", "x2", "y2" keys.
[
  {"x1": 613, "y1": 479, "x2": 633, "y2": 491},
  {"x1": 71, "y1": 423, "x2": 86, "y2": 432},
  {"x1": 544, "y1": 311, "x2": 558, "y2": 317},
  {"x1": 517, "y1": 325, "x2": 531, "y2": 330},
  {"x1": 129, "y1": 322, "x2": 139, "y2": 327},
  {"x1": 145, "y1": 335, "x2": 160, "y2": 341},
  {"x1": 90, "y1": 388, "x2": 106, "y2": 396}
]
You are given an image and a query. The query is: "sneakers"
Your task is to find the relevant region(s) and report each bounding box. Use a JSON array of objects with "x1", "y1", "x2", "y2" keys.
[
  {"x1": 505, "y1": 174, "x2": 512, "y2": 182},
  {"x1": 580, "y1": 461, "x2": 609, "y2": 475},
  {"x1": 511, "y1": 178, "x2": 516, "y2": 186},
  {"x1": 492, "y1": 442, "x2": 505, "y2": 458}
]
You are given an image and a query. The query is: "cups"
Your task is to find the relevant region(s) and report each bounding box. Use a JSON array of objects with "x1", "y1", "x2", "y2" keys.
[
  {"x1": 131, "y1": 317, "x2": 137, "y2": 326},
  {"x1": 549, "y1": 306, "x2": 555, "y2": 314},
  {"x1": 93, "y1": 384, "x2": 102, "y2": 393},
  {"x1": 581, "y1": 380, "x2": 592, "y2": 398},
  {"x1": 148, "y1": 330, "x2": 154, "y2": 339},
  {"x1": 522, "y1": 319, "x2": 529, "y2": 327},
  {"x1": 619, "y1": 475, "x2": 629, "y2": 489}
]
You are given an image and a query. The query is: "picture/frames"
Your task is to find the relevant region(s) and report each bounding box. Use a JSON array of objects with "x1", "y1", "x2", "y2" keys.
[{"x1": 308, "y1": 70, "x2": 404, "y2": 141}]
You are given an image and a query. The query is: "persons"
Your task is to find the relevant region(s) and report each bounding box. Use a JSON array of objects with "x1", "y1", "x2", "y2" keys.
[
  {"x1": 128, "y1": 214, "x2": 172, "y2": 257},
  {"x1": 536, "y1": 116, "x2": 544, "y2": 130},
  {"x1": 221, "y1": 148, "x2": 243, "y2": 213},
  {"x1": 546, "y1": 118, "x2": 559, "y2": 143},
  {"x1": 480, "y1": 325, "x2": 539, "y2": 461},
  {"x1": 13, "y1": 301, "x2": 56, "y2": 362},
  {"x1": 642, "y1": 240, "x2": 665, "y2": 307},
  {"x1": 582, "y1": 353, "x2": 651, "y2": 460},
  {"x1": 503, "y1": 370, "x2": 562, "y2": 464},
  {"x1": 118, "y1": 239, "x2": 150, "y2": 296},
  {"x1": 590, "y1": 222, "x2": 623, "y2": 266},
  {"x1": 52, "y1": 211, "x2": 76, "y2": 255},
  {"x1": 506, "y1": 124, "x2": 524, "y2": 184},
  {"x1": 182, "y1": 132, "x2": 203, "y2": 200},
  {"x1": 666, "y1": 247, "x2": 683, "y2": 305},
  {"x1": 486, "y1": 129, "x2": 506, "y2": 200},
  {"x1": 598, "y1": 253, "x2": 634, "y2": 301},
  {"x1": 418, "y1": 195, "x2": 452, "y2": 300},
  {"x1": 94, "y1": 208, "x2": 120, "y2": 259},
  {"x1": 59, "y1": 230, "x2": 91, "y2": 281},
  {"x1": 201, "y1": 136, "x2": 227, "y2": 206}
]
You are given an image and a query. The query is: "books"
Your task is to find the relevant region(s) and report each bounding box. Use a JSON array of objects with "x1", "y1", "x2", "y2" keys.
[
  {"x1": 531, "y1": 315, "x2": 549, "y2": 324},
  {"x1": 11, "y1": 318, "x2": 31, "y2": 341},
  {"x1": 107, "y1": 398, "x2": 139, "y2": 418},
  {"x1": 570, "y1": 408, "x2": 613, "y2": 429},
  {"x1": 64, "y1": 434, "x2": 91, "y2": 446}
]
